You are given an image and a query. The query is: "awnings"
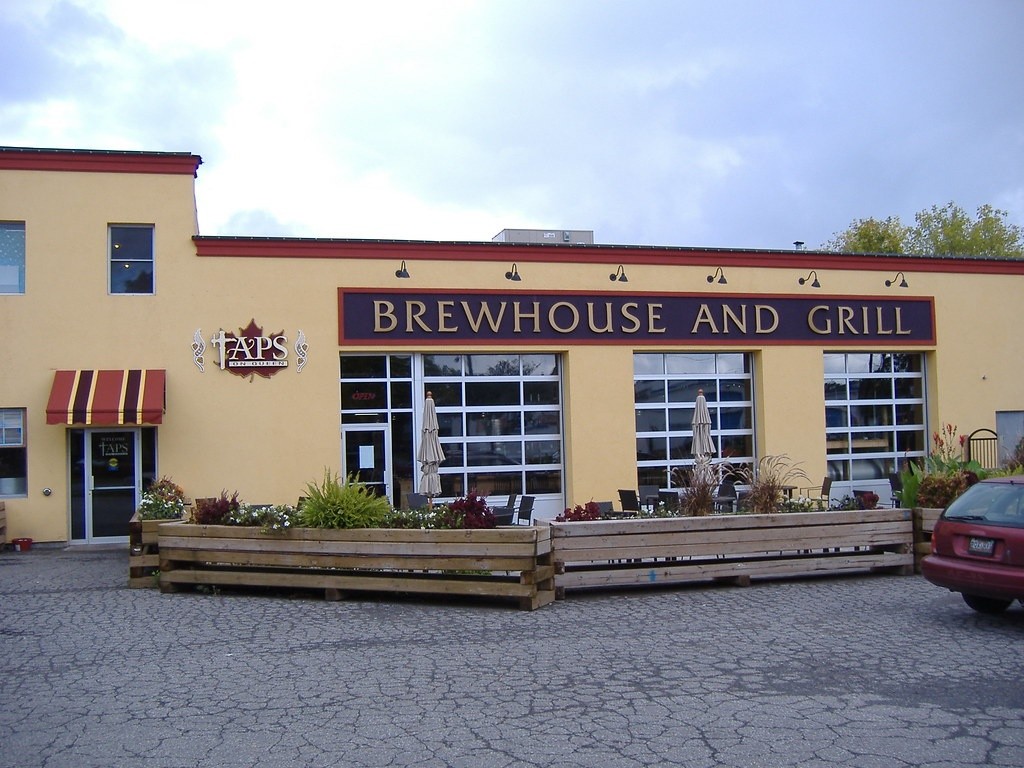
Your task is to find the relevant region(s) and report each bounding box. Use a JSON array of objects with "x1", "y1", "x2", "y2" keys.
[{"x1": 45, "y1": 369, "x2": 166, "y2": 426}]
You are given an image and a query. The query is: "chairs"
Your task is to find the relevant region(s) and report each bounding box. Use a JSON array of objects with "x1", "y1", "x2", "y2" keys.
[
  {"x1": 800, "y1": 476, "x2": 833, "y2": 509},
  {"x1": 716, "y1": 483, "x2": 738, "y2": 515},
  {"x1": 853, "y1": 490, "x2": 883, "y2": 510},
  {"x1": 406, "y1": 491, "x2": 536, "y2": 526},
  {"x1": 595, "y1": 484, "x2": 686, "y2": 520},
  {"x1": 888, "y1": 472, "x2": 904, "y2": 508}
]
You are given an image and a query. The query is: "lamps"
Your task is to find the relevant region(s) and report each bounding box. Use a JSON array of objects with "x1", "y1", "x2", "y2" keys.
[
  {"x1": 609, "y1": 265, "x2": 628, "y2": 282},
  {"x1": 395, "y1": 260, "x2": 410, "y2": 278},
  {"x1": 504, "y1": 263, "x2": 521, "y2": 281},
  {"x1": 707, "y1": 267, "x2": 727, "y2": 284},
  {"x1": 885, "y1": 272, "x2": 908, "y2": 288},
  {"x1": 799, "y1": 271, "x2": 820, "y2": 287}
]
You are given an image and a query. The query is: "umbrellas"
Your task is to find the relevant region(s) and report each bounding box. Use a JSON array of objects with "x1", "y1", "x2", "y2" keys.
[
  {"x1": 415, "y1": 391, "x2": 446, "y2": 511},
  {"x1": 690, "y1": 389, "x2": 716, "y2": 495}
]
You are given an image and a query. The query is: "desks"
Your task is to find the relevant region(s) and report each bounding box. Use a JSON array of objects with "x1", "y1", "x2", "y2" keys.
[
  {"x1": 771, "y1": 486, "x2": 798, "y2": 505},
  {"x1": 646, "y1": 495, "x2": 685, "y2": 516},
  {"x1": 712, "y1": 497, "x2": 737, "y2": 513}
]
